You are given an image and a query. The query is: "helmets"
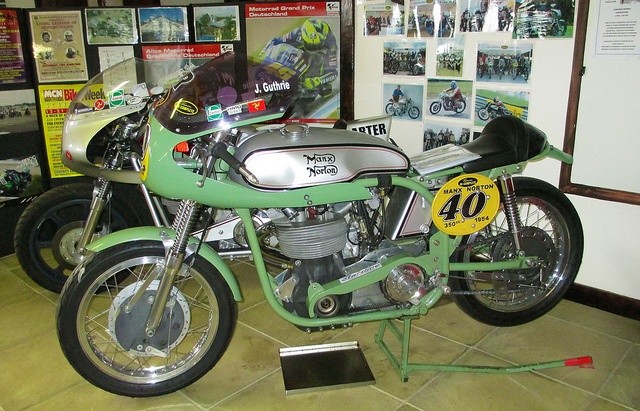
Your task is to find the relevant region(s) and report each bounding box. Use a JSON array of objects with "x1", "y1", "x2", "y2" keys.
[
  {"x1": 451, "y1": 81, "x2": 457, "y2": 88},
  {"x1": 494, "y1": 96, "x2": 499, "y2": 102},
  {"x1": 302, "y1": 18, "x2": 329, "y2": 46}
]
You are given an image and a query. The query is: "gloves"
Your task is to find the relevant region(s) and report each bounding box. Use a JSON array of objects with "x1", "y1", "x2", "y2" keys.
[{"x1": 304, "y1": 76, "x2": 321, "y2": 89}]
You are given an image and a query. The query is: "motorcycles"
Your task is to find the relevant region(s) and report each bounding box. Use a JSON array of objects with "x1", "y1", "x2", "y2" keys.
[
  {"x1": 430, "y1": 90, "x2": 466, "y2": 114},
  {"x1": 478, "y1": 60, "x2": 528, "y2": 81},
  {"x1": 385, "y1": 98, "x2": 420, "y2": 119},
  {"x1": 478, "y1": 100, "x2": 512, "y2": 121},
  {"x1": 53, "y1": 53, "x2": 585, "y2": 399},
  {"x1": 13, "y1": 52, "x2": 393, "y2": 296},
  {"x1": 255, "y1": 43, "x2": 338, "y2": 119}
]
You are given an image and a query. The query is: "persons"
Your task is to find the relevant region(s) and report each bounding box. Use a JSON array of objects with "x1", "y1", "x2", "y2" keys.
[
  {"x1": 460, "y1": 6, "x2": 510, "y2": 31},
  {"x1": 408, "y1": 13, "x2": 453, "y2": 36},
  {"x1": 424, "y1": 127, "x2": 474, "y2": 151},
  {"x1": 476, "y1": 52, "x2": 530, "y2": 79},
  {"x1": 268, "y1": 17, "x2": 339, "y2": 97},
  {"x1": 365, "y1": 10, "x2": 403, "y2": 36},
  {"x1": 383, "y1": 47, "x2": 425, "y2": 75},
  {"x1": 491, "y1": 97, "x2": 506, "y2": 107},
  {"x1": 438, "y1": 54, "x2": 462, "y2": 71},
  {"x1": 392, "y1": 84, "x2": 404, "y2": 103},
  {"x1": 446, "y1": 81, "x2": 463, "y2": 107}
]
